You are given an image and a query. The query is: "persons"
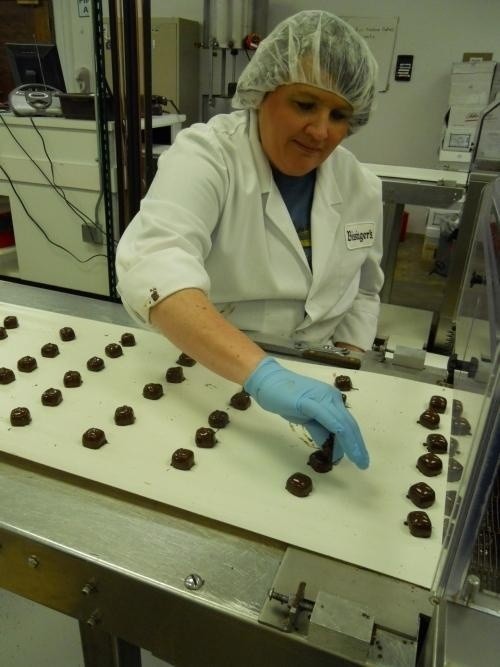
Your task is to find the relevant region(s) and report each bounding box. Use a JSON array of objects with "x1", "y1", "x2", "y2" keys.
[{"x1": 114, "y1": 7, "x2": 385, "y2": 472}]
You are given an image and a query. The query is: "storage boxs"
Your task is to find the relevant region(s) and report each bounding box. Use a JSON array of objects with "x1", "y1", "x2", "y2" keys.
[{"x1": 422, "y1": 58, "x2": 497, "y2": 261}]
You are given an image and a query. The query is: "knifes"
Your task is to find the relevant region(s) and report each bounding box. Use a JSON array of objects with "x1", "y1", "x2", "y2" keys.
[{"x1": 255, "y1": 339, "x2": 361, "y2": 370}]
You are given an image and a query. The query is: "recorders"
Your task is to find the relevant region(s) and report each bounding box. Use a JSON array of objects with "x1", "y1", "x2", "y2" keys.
[{"x1": 8, "y1": 83, "x2": 64, "y2": 117}]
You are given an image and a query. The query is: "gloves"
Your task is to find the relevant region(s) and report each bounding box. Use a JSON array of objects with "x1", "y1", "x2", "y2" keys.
[{"x1": 244, "y1": 356, "x2": 369, "y2": 470}]
